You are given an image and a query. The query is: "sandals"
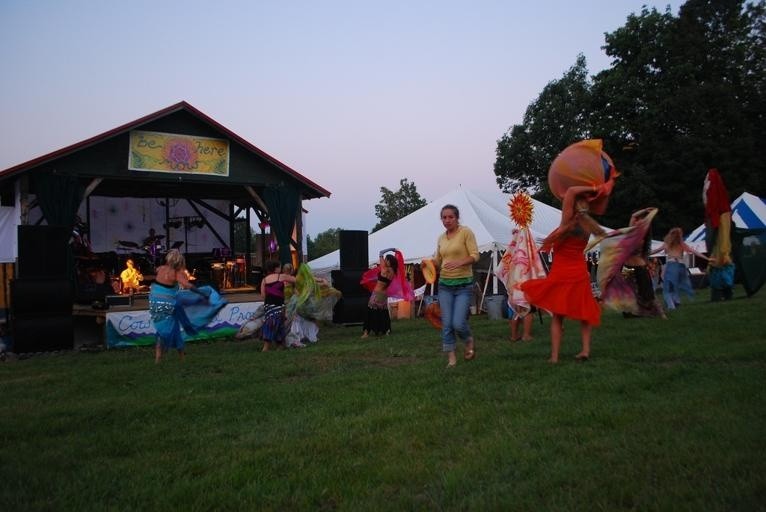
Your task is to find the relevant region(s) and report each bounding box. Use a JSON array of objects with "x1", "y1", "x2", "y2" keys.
[
  {"x1": 464, "y1": 341, "x2": 475, "y2": 361},
  {"x1": 510, "y1": 334, "x2": 521, "y2": 342},
  {"x1": 573, "y1": 350, "x2": 590, "y2": 362},
  {"x1": 521, "y1": 334, "x2": 534, "y2": 342},
  {"x1": 446, "y1": 359, "x2": 458, "y2": 370}
]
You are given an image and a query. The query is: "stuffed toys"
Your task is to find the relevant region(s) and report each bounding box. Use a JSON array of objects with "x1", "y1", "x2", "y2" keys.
[{"x1": 702, "y1": 168, "x2": 736, "y2": 301}]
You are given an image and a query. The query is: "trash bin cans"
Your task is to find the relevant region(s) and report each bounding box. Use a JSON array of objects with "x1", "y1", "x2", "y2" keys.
[{"x1": 483, "y1": 292, "x2": 508, "y2": 321}]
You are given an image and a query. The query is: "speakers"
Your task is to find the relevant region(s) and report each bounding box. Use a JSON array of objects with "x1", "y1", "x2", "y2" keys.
[
  {"x1": 16, "y1": 224, "x2": 71, "y2": 278},
  {"x1": 339, "y1": 229, "x2": 370, "y2": 272}
]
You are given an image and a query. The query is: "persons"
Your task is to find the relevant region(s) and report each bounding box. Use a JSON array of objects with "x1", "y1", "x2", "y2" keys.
[
  {"x1": 71, "y1": 230, "x2": 149, "y2": 304},
  {"x1": 148, "y1": 248, "x2": 211, "y2": 365},
  {"x1": 235, "y1": 260, "x2": 342, "y2": 353},
  {"x1": 420, "y1": 205, "x2": 481, "y2": 368},
  {"x1": 361, "y1": 248, "x2": 416, "y2": 339},
  {"x1": 496, "y1": 186, "x2": 708, "y2": 364}
]
